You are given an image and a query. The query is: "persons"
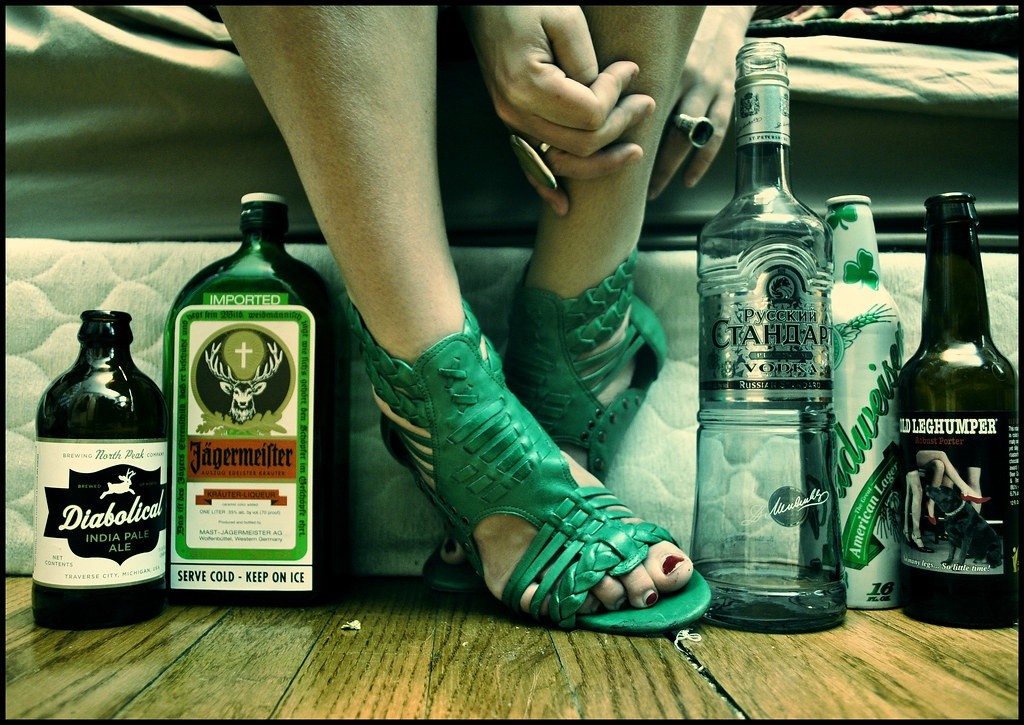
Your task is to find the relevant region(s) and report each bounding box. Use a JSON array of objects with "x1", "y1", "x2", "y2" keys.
[{"x1": 218, "y1": 5, "x2": 757, "y2": 635}]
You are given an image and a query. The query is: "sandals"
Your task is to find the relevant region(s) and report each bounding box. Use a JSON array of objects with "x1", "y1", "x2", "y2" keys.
[
  {"x1": 349, "y1": 297, "x2": 711, "y2": 635},
  {"x1": 504, "y1": 247, "x2": 667, "y2": 484}
]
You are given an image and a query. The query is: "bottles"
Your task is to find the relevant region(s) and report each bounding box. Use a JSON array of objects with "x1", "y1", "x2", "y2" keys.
[
  {"x1": 165, "y1": 191, "x2": 334, "y2": 610},
  {"x1": 897, "y1": 193, "x2": 1018, "y2": 629},
  {"x1": 31, "y1": 307, "x2": 169, "y2": 632},
  {"x1": 691, "y1": 41, "x2": 848, "y2": 635},
  {"x1": 823, "y1": 195, "x2": 905, "y2": 610}
]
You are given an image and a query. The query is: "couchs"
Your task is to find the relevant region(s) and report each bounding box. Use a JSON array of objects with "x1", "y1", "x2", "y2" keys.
[{"x1": 6, "y1": 5, "x2": 1019, "y2": 575}]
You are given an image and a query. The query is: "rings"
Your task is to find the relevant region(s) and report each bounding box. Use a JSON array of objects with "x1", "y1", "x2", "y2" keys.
[
  {"x1": 672, "y1": 114, "x2": 715, "y2": 148},
  {"x1": 510, "y1": 134, "x2": 558, "y2": 191}
]
